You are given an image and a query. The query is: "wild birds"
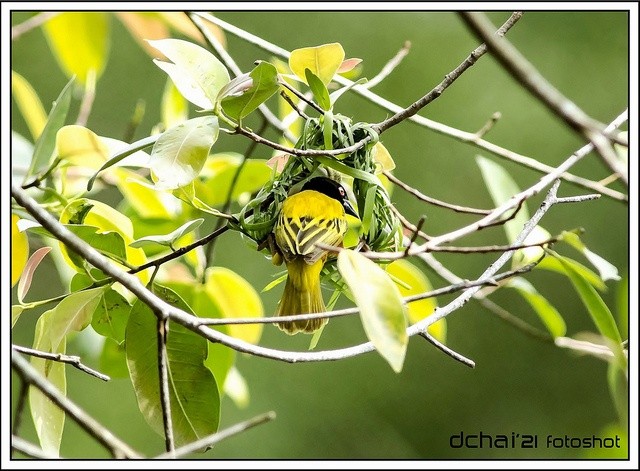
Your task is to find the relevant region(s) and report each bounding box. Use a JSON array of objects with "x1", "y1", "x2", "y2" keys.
[{"x1": 256, "y1": 176, "x2": 361, "y2": 337}]
